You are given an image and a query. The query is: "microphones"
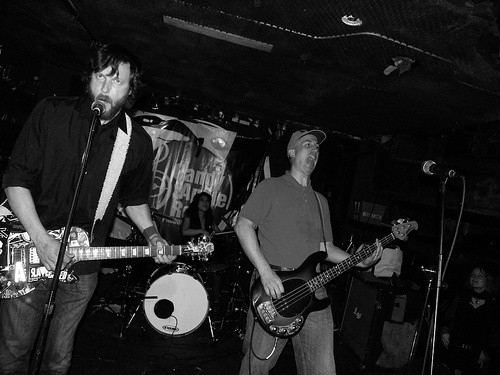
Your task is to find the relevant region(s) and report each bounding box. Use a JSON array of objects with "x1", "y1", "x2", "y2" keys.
[
  {"x1": 420, "y1": 161, "x2": 457, "y2": 177},
  {"x1": 91, "y1": 100, "x2": 106, "y2": 116}
]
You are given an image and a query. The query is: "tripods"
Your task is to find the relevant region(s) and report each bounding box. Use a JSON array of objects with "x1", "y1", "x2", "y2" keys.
[
  {"x1": 86, "y1": 265, "x2": 146, "y2": 340},
  {"x1": 226, "y1": 251, "x2": 248, "y2": 313}
]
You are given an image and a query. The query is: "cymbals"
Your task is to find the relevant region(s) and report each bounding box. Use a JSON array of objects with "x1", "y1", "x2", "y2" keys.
[{"x1": 154, "y1": 212, "x2": 175, "y2": 222}]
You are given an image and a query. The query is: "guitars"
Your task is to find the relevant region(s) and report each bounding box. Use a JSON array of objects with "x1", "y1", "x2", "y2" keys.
[
  {"x1": 0, "y1": 226, "x2": 215, "y2": 299},
  {"x1": 249, "y1": 217, "x2": 419, "y2": 340}
]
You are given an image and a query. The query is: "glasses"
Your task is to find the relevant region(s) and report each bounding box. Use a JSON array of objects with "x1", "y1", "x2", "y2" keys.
[{"x1": 470, "y1": 272, "x2": 485, "y2": 280}]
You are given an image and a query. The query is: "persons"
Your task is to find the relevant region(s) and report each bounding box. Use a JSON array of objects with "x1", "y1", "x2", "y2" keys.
[
  {"x1": 0, "y1": 33, "x2": 177, "y2": 375},
  {"x1": 440, "y1": 262, "x2": 499, "y2": 373},
  {"x1": 181, "y1": 191, "x2": 222, "y2": 244},
  {"x1": 235, "y1": 128, "x2": 384, "y2": 375}
]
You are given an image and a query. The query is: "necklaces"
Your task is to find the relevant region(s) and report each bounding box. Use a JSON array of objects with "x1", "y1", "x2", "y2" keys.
[{"x1": 474, "y1": 297, "x2": 482, "y2": 304}]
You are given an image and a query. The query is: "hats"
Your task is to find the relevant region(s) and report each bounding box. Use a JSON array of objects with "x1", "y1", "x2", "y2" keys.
[{"x1": 286, "y1": 130, "x2": 326, "y2": 159}]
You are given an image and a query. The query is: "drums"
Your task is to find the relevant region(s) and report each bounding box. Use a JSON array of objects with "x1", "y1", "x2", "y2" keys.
[{"x1": 143, "y1": 262, "x2": 209, "y2": 338}]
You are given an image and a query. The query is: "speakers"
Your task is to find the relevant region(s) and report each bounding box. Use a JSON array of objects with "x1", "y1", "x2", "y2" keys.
[{"x1": 338, "y1": 276, "x2": 415, "y2": 365}]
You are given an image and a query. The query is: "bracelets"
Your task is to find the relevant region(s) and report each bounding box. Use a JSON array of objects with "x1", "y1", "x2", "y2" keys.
[{"x1": 142, "y1": 224, "x2": 159, "y2": 242}]
